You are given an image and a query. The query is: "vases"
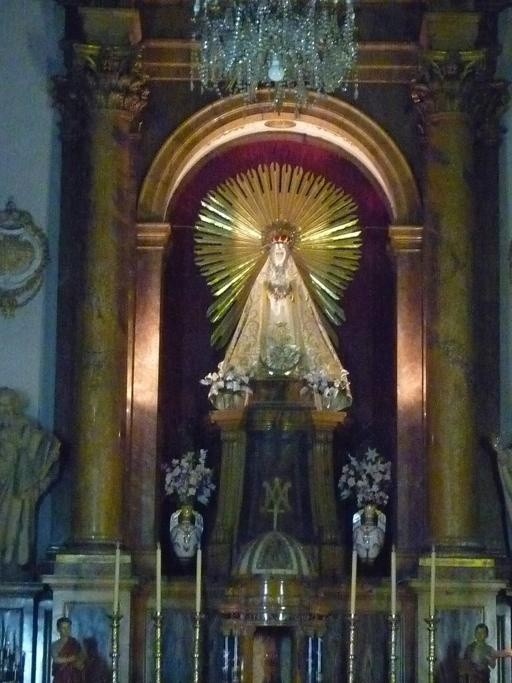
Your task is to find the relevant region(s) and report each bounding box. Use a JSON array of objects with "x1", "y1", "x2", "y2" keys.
[
  {"x1": 351, "y1": 512, "x2": 387, "y2": 564},
  {"x1": 171, "y1": 509, "x2": 202, "y2": 562}
]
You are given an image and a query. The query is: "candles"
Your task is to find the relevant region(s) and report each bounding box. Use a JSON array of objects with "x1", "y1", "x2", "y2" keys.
[
  {"x1": 428, "y1": 545, "x2": 437, "y2": 616},
  {"x1": 112, "y1": 542, "x2": 121, "y2": 614},
  {"x1": 350, "y1": 544, "x2": 357, "y2": 615},
  {"x1": 391, "y1": 544, "x2": 397, "y2": 615},
  {"x1": 154, "y1": 541, "x2": 161, "y2": 614},
  {"x1": 194, "y1": 543, "x2": 203, "y2": 615}
]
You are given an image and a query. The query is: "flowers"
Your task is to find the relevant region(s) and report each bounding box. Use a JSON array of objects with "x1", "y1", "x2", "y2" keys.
[
  {"x1": 338, "y1": 449, "x2": 392, "y2": 512},
  {"x1": 163, "y1": 449, "x2": 214, "y2": 507}
]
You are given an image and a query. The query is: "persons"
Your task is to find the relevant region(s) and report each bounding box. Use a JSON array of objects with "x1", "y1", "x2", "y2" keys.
[
  {"x1": 458, "y1": 622, "x2": 496, "y2": 683},
  {"x1": 51, "y1": 617, "x2": 89, "y2": 683}
]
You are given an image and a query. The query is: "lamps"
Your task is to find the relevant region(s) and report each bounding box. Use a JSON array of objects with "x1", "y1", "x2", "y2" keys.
[{"x1": 186, "y1": 0, "x2": 360, "y2": 116}]
